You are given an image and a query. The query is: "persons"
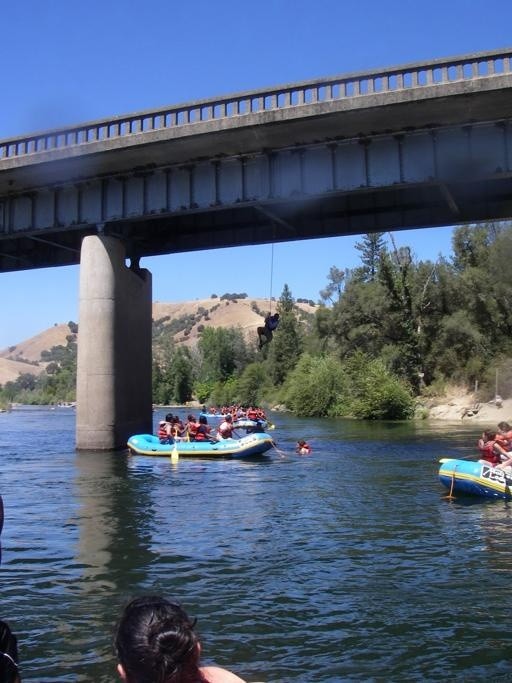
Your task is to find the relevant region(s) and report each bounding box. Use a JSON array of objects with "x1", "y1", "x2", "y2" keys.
[
  {"x1": 113, "y1": 595, "x2": 246, "y2": 683},
  {"x1": 478, "y1": 430, "x2": 512, "y2": 469},
  {"x1": 257, "y1": 312, "x2": 280, "y2": 350},
  {"x1": 495, "y1": 421, "x2": 512, "y2": 452},
  {"x1": 157, "y1": 403, "x2": 267, "y2": 444},
  {"x1": 296, "y1": 440, "x2": 311, "y2": 455}
]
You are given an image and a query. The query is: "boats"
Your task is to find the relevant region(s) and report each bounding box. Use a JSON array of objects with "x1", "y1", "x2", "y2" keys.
[
  {"x1": 126, "y1": 432, "x2": 277, "y2": 459},
  {"x1": 231, "y1": 418, "x2": 267, "y2": 431},
  {"x1": 439, "y1": 458, "x2": 512, "y2": 507},
  {"x1": 199, "y1": 410, "x2": 234, "y2": 420},
  {"x1": 126, "y1": 454, "x2": 270, "y2": 475}
]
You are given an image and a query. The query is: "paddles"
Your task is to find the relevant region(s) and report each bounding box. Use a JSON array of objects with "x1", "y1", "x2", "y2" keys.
[
  {"x1": 439, "y1": 453, "x2": 481, "y2": 463},
  {"x1": 259, "y1": 414, "x2": 275, "y2": 429},
  {"x1": 171, "y1": 429, "x2": 179, "y2": 465}
]
